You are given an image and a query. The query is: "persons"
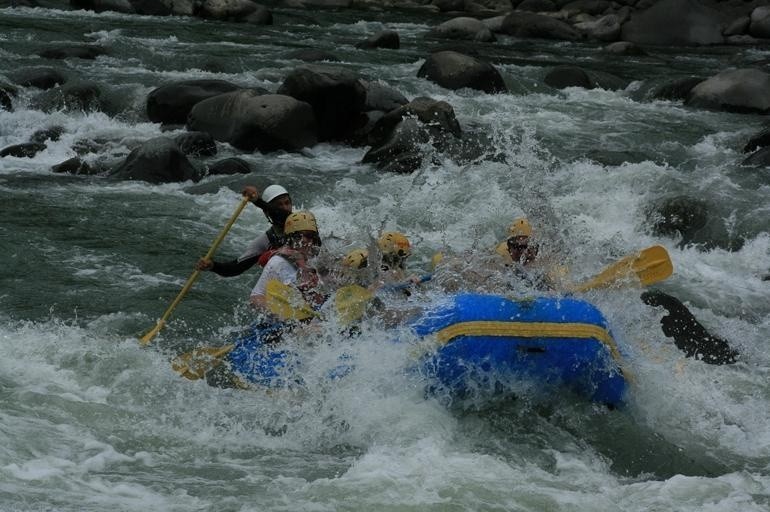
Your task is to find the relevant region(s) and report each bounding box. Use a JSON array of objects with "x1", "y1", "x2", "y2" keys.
[
  {"x1": 496, "y1": 217, "x2": 541, "y2": 276},
  {"x1": 199, "y1": 183, "x2": 295, "y2": 278},
  {"x1": 319, "y1": 232, "x2": 420, "y2": 298},
  {"x1": 246, "y1": 211, "x2": 321, "y2": 308}
]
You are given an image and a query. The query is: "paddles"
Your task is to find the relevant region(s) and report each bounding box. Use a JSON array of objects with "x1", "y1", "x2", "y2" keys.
[
  {"x1": 334, "y1": 271, "x2": 433, "y2": 325},
  {"x1": 563, "y1": 244, "x2": 674, "y2": 297},
  {"x1": 172, "y1": 326, "x2": 287, "y2": 380},
  {"x1": 267, "y1": 280, "x2": 331, "y2": 323}
]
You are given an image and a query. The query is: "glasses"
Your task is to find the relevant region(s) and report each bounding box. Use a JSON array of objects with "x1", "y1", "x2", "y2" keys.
[
  {"x1": 509, "y1": 242, "x2": 528, "y2": 251},
  {"x1": 288, "y1": 232, "x2": 315, "y2": 243}
]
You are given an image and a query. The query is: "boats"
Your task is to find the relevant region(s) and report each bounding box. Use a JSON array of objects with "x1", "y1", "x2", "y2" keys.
[{"x1": 231, "y1": 292, "x2": 632, "y2": 409}]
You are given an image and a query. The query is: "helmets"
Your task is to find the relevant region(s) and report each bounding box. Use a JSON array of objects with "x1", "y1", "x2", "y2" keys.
[
  {"x1": 342, "y1": 248, "x2": 369, "y2": 278},
  {"x1": 261, "y1": 183, "x2": 288, "y2": 203},
  {"x1": 282, "y1": 210, "x2": 320, "y2": 237},
  {"x1": 511, "y1": 217, "x2": 532, "y2": 239},
  {"x1": 378, "y1": 232, "x2": 413, "y2": 262}
]
